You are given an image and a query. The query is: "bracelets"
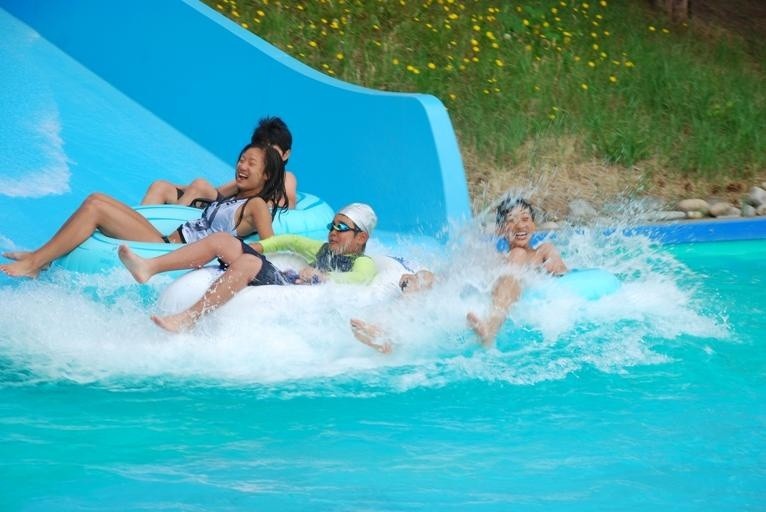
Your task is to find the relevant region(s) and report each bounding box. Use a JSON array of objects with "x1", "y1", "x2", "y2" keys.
[{"x1": 311, "y1": 272, "x2": 319, "y2": 284}]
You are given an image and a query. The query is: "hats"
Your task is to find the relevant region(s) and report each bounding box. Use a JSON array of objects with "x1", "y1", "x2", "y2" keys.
[{"x1": 338, "y1": 201, "x2": 379, "y2": 238}]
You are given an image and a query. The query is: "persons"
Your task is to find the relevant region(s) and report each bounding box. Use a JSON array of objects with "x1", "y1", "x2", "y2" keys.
[
  {"x1": 348, "y1": 197, "x2": 569, "y2": 356},
  {"x1": 117, "y1": 201, "x2": 381, "y2": 337},
  {"x1": 0, "y1": 141, "x2": 290, "y2": 279},
  {"x1": 141, "y1": 116, "x2": 297, "y2": 212}
]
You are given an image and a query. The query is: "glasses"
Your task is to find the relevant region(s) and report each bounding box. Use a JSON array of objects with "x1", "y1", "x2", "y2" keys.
[{"x1": 326, "y1": 221, "x2": 362, "y2": 234}]
